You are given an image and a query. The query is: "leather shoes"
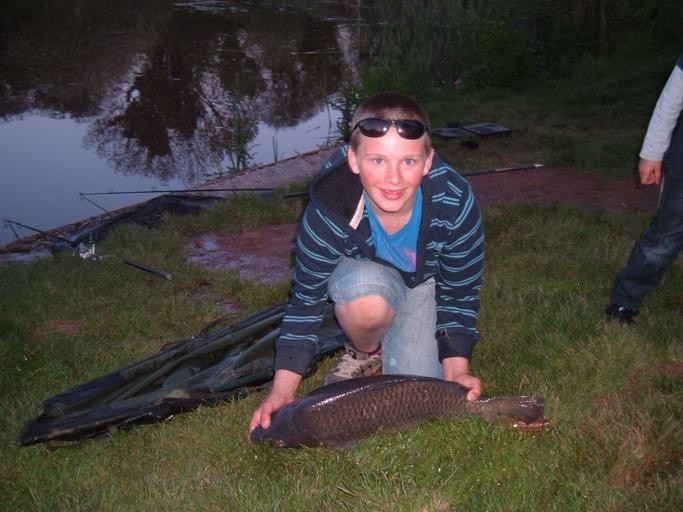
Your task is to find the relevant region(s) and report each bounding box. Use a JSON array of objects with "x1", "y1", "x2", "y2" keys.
[{"x1": 605, "y1": 303, "x2": 639, "y2": 325}]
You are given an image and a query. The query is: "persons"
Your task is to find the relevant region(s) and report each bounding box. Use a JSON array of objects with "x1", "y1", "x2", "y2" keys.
[
  {"x1": 248, "y1": 92, "x2": 485, "y2": 444},
  {"x1": 602, "y1": 56, "x2": 683, "y2": 326}
]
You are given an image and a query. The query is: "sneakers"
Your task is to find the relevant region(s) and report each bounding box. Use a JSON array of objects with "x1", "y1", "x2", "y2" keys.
[{"x1": 322, "y1": 337, "x2": 382, "y2": 387}]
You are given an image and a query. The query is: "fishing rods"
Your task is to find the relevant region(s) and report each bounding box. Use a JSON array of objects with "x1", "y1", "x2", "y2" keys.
[
  {"x1": 284, "y1": 163, "x2": 543, "y2": 199},
  {"x1": 4, "y1": 218, "x2": 173, "y2": 283},
  {"x1": 77, "y1": 185, "x2": 285, "y2": 217}
]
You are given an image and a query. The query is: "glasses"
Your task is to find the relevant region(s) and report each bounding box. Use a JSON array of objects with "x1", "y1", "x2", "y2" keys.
[{"x1": 346, "y1": 116, "x2": 433, "y2": 144}]
